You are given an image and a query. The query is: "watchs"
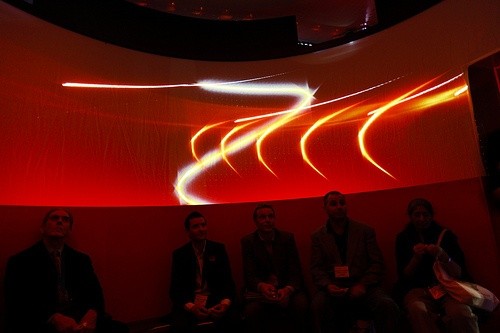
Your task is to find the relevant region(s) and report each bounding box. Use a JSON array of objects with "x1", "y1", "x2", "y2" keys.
[{"x1": 445, "y1": 257, "x2": 452, "y2": 267}]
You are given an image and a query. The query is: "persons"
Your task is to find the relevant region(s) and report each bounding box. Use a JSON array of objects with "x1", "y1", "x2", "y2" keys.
[
  {"x1": 394, "y1": 197, "x2": 479, "y2": 333},
  {"x1": 310, "y1": 190, "x2": 398, "y2": 333},
  {"x1": 1, "y1": 207, "x2": 113, "y2": 333},
  {"x1": 167, "y1": 209, "x2": 239, "y2": 333},
  {"x1": 240, "y1": 205, "x2": 308, "y2": 333}
]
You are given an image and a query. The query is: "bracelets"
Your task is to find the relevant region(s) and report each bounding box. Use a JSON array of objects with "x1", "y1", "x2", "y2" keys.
[{"x1": 283, "y1": 286, "x2": 293, "y2": 294}]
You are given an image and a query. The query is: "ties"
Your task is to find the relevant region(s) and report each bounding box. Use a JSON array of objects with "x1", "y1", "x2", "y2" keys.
[{"x1": 53, "y1": 255, "x2": 68, "y2": 305}]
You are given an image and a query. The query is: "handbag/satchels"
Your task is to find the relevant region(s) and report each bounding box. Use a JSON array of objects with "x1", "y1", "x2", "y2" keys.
[{"x1": 433, "y1": 229, "x2": 500, "y2": 311}]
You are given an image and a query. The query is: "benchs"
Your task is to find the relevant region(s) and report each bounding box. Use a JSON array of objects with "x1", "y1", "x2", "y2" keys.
[{"x1": 128, "y1": 300, "x2": 445, "y2": 333}]
